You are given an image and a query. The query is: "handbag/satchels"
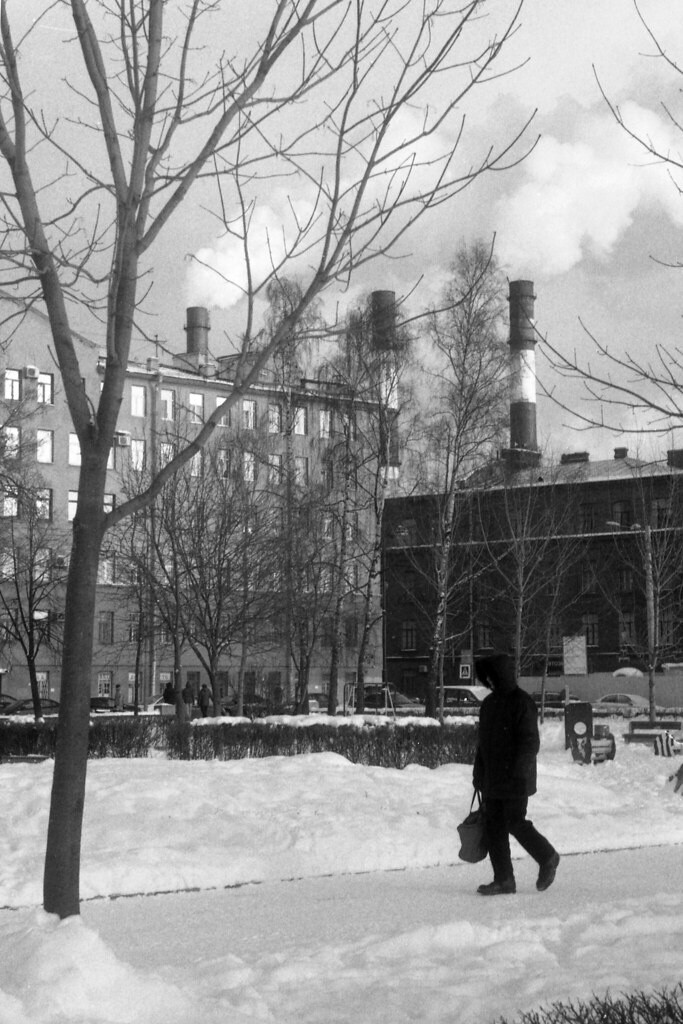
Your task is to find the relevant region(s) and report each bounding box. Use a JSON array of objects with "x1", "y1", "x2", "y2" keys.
[{"x1": 456, "y1": 789, "x2": 488, "y2": 863}]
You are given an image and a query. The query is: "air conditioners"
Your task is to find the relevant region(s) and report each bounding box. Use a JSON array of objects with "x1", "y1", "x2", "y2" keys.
[
  {"x1": 56, "y1": 613, "x2": 66, "y2": 622},
  {"x1": 117, "y1": 435, "x2": 130, "y2": 446},
  {"x1": 23, "y1": 367, "x2": 39, "y2": 379},
  {"x1": 54, "y1": 555, "x2": 69, "y2": 567}
]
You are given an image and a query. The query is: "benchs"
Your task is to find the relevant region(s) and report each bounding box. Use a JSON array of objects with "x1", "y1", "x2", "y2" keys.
[{"x1": 623, "y1": 721, "x2": 683, "y2": 746}]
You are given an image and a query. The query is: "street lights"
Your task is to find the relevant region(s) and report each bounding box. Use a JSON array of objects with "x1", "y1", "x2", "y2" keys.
[{"x1": 606, "y1": 521, "x2": 656, "y2": 666}]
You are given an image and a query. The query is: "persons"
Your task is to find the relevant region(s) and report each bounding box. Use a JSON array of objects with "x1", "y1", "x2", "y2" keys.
[
  {"x1": 197, "y1": 684, "x2": 214, "y2": 717},
  {"x1": 182, "y1": 682, "x2": 194, "y2": 719},
  {"x1": 471, "y1": 653, "x2": 560, "y2": 895},
  {"x1": 114, "y1": 685, "x2": 125, "y2": 711},
  {"x1": 163, "y1": 682, "x2": 177, "y2": 706}
]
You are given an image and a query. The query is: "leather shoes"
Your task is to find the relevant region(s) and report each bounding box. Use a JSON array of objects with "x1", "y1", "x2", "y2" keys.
[
  {"x1": 477, "y1": 877, "x2": 516, "y2": 894},
  {"x1": 535, "y1": 851, "x2": 560, "y2": 891}
]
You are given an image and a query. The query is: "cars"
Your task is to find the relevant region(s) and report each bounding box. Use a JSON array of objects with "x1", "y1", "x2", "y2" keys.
[
  {"x1": 223, "y1": 695, "x2": 273, "y2": 717},
  {"x1": 595, "y1": 694, "x2": 650, "y2": 708},
  {"x1": 191, "y1": 698, "x2": 227, "y2": 718},
  {"x1": 348, "y1": 688, "x2": 427, "y2": 708},
  {"x1": 422, "y1": 685, "x2": 492, "y2": 714},
  {"x1": 1, "y1": 694, "x2": 25, "y2": 715},
  {"x1": 3, "y1": 696, "x2": 61, "y2": 716},
  {"x1": 287, "y1": 692, "x2": 339, "y2": 716},
  {"x1": 125, "y1": 693, "x2": 176, "y2": 713},
  {"x1": 92, "y1": 698, "x2": 140, "y2": 714}
]
